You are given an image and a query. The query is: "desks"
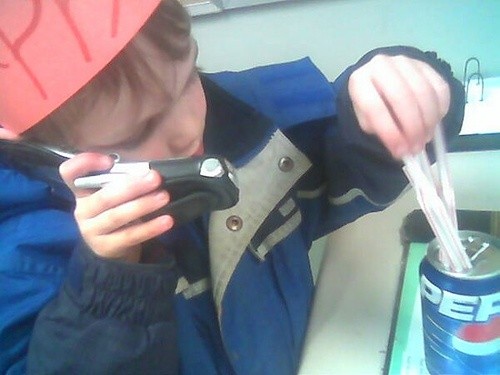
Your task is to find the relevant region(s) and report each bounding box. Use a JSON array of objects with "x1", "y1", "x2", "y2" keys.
[{"x1": 297, "y1": 150, "x2": 500, "y2": 375}]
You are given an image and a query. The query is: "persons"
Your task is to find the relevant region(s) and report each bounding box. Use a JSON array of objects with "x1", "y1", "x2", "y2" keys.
[{"x1": 0, "y1": 1, "x2": 465, "y2": 374}]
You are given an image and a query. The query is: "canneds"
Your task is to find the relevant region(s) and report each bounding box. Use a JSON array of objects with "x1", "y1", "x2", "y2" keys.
[{"x1": 419, "y1": 230, "x2": 500, "y2": 375}]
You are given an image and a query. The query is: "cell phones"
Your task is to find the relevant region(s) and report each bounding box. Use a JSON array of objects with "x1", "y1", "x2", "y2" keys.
[{"x1": 3, "y1": 140, "x2": 240, "y2": 226}]
